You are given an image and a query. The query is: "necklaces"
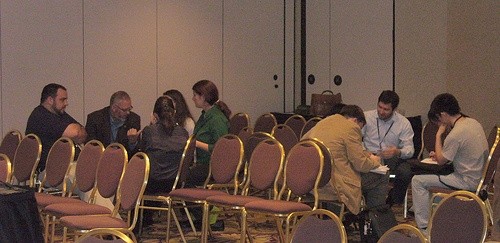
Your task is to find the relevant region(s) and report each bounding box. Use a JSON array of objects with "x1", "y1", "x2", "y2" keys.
[{"x1": 453, "y1": 116, "x2": 462, "y2": 127}]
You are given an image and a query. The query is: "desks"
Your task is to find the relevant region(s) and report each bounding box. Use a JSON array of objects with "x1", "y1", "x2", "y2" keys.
[
  {"x1": 271, "y1": 111, "x2": 423, "y2": 205},
  {"x1": 0, "y1": 180, "x2": 47, "y2": 243}
]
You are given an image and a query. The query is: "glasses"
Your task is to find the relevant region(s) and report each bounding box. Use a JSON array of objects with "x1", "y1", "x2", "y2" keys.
[{"x1": 116, "y1": 104, "x2": 133, "y2": 112}]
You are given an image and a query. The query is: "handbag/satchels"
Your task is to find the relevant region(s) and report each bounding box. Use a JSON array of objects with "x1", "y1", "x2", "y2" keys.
[
  {"x1": 359, "y1": 205, "x2": 397, "y2": 243},
  {"x1": 410, "y1": 162, "x2": 454, "y2": 175}
]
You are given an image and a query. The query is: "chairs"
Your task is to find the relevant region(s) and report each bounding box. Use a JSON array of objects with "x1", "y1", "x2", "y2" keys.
[
  {"x1": 0, "y1": 95, "x2": 353, "y2": 243},
  {"x1": 374, "y1": 116, "x2": 500, "y2": 243}
]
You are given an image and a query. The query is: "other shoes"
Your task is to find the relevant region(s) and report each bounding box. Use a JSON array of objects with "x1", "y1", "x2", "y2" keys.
[
  {"x1": 193, "y1": 220, "x2": 224, "y2": 231},
  {"x1": 406, "y1": 208, "x2": 416, "y2": 219},
  {"x1": 386, "y1": 197, "x2": 394, "y2": 208}
]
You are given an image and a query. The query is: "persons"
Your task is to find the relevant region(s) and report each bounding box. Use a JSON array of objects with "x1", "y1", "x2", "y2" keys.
[
  {"x1": 412, "y1": 93, "x2": 489, "y2": 233},
  {"x1": 301, "y1": 105, "x2": 389, "y2": 216},
  {"x1": 26, "y1": 83, "x2": 122, "y2": 220},
  {"x1": 131, "y1": 97, "x2": 189, "y2": 233},
  {"x1": 360, "y1": 90, "x2": 415, "y2": 211},
  {"x1": 85, "y1": 91, "x2": 142, "y2": 157},
  {"x1": 179, "y1": 80, "x2": 230, "y2": 231},
  {"x1": 163, "y1": 90, "x2": 195, "y2": 133}
]
